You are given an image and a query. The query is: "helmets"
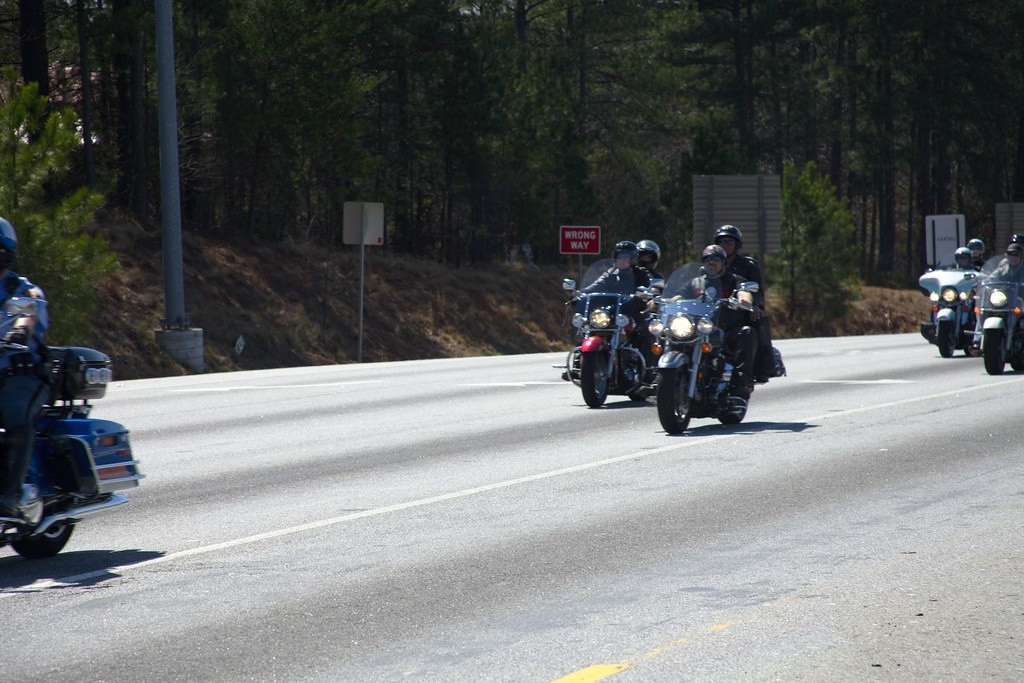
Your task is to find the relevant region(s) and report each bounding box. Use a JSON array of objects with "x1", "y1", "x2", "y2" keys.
[
  {"x1": 955, "y1": 247, "x2": 972, "y2": 262},
  {"x1": 613, "y1": 241, "x2": 639, "y2": 268},
  {"x1": 700, "y1": 245, "x2": 727, "y2": 260},
  {"x1": 0, "y1": 215, "x2": 17, "y2": 253},
  {"x1": 714, "y1": 224, "x2": 743, "y2": 246},
  {"x1": 636, "y1": 239, "x2": 660, "y2": 271},
  {"x1": 1007, "y1": 243, "x2": 1022, "y2": 255},
  {"x1": 967, "y1": 238, "x2": 985, "y2": 253},
  {"x1": 1011, "y1": 234, "x2": 1024, "y2": 245}
]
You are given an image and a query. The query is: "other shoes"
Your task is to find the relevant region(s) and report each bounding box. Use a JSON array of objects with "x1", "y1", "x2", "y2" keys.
[
  {"x1": 737, "y1": 384, "x2": 750, "y2": 399},
  {"x1": 563, "y1": 363, "x2": 581, "y2": 381},
  {"x1": 639, "y1": 384, "x2": 656, "y2": 400}
]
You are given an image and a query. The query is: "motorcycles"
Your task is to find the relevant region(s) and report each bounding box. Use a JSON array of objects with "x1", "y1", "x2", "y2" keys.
[
  {"x1": 0, "y1": 298, "x2": 144, "y2": 561},
  {"x1": 916, "y1": 249, "x2": 988, "y2": 359},
  {"x1": 963, "y1": 254, "x2": 1024, "y2": 375},
  {"x1": 640, "y1": 261, "x2": 788, "y2": 436},
  {"x1": 553, "y1": 261, "x2": 665, "y2": 409}
]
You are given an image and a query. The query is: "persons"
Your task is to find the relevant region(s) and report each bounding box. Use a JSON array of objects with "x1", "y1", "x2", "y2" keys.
[
  {"x1": 0, "y1": 217, "x2": 49, "y2": 521},
  {"x1": 920, "y1": 238, "x2": 992, "y2": 336},
  {"x1": 648, "y1": 224, "x2": 765, "y2": 400},
  {"x1": 562, "y1": 240, "x2": 666, "y2": 381},
  {"x1": 984, "y1": 234, "x2": 1024, "y2": 306}
]
